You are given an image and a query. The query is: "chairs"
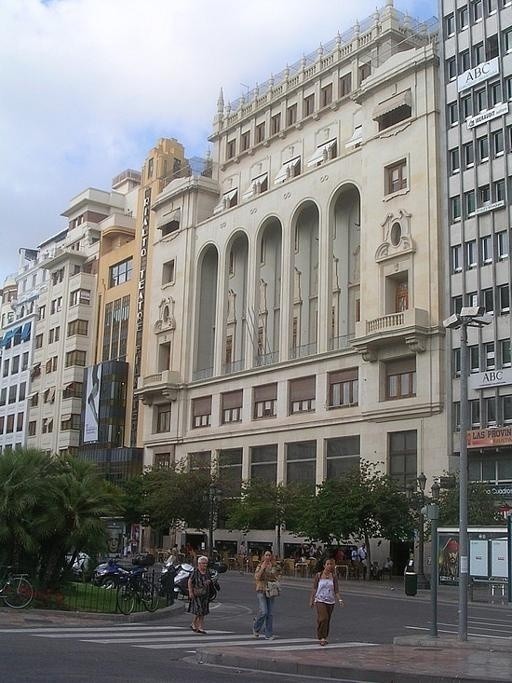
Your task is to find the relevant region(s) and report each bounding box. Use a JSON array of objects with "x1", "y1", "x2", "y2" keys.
[{"x1": 154, "y1": 546, "x2": 393, "y2": 579}]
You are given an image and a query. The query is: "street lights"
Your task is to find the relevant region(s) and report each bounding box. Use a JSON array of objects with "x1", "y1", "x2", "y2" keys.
[
  {"x1": 406, "y1": 471, "x2": 429, "y2": 589},
  {"x1": 270, "y1": 486, "x2": 291, "y2": 563},
  {"x1": 427, "y1": 481, "x2": 442, "y2": 636},
  {"x1": 200, "y1": 479, "x2": 225, "y2": 562}
]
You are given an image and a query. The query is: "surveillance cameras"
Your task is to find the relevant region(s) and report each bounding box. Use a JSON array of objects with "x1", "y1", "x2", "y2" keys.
[
  {"x1": 460, "y1": 306, "x2": 486, "y2": 318},
  {"x1": 443, "y1": 313, "x2": 463, "y2": 329},
  {"x1": 472, "y1": 313, "x2": 492, "y2": 325}
]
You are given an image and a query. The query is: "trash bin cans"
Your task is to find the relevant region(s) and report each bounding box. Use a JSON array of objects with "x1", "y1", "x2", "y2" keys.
[{"x1": 404, "y1": 572, "x2": 417, "y2": 597}]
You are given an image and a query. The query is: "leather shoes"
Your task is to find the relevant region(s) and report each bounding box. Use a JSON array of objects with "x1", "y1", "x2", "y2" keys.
[
  {"x1": 191, "y1": 625, "x2": 197, "y2": 631},
  {"x1": 198, "y1": 630, "x2": 206, "y2": 635}
]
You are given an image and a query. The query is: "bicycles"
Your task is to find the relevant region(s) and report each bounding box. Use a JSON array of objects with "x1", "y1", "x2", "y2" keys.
[
  {"x1": 0, "y1": 564, "x2": 31, "y2": 608},
  {"x1": 110, "y1": 570, "x2": 161, "y2": 614}
]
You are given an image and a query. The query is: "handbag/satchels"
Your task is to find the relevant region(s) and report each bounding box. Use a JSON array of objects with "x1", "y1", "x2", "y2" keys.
[
  {"x1": 193, "y1": 587, "x2": 206, "y2": 596},
  {"x1": 266, "y1": 581, "x2": 279, "y2": 598}
]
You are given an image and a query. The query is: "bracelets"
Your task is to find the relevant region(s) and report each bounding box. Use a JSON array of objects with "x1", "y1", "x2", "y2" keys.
[{"x1": 338, "y1": 598, "x2": 343, "y2": 603}]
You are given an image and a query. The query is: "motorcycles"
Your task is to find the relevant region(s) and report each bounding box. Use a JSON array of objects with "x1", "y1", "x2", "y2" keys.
[{"x1": 157, "y1": 549, "x2": 227, "y2": 602}]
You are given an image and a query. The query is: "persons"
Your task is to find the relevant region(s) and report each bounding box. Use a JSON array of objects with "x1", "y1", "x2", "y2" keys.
[
  {"x1": 405, "y1": 552, "x2": 416, "y2": 573},
  {"x1": 188, "y1": 556, "x2": 217, "y2": 633},
  {"x1": 251, "y1": 548, "x2": 283, "y2": 640},
  {"x1": 122, "y1": 532, "x2": 393, "y2": 579},
  {"x1": 439, "y1": 538, "x2": 458, "y2": 577},
  {"x1": 86, "y1": 365, "x2": 101, "y2": 425},
  {"x1": 308, "y1": 556, "x2": 345, "y2": 645},
  {"x1": 105, "y1": 525, "x2": 123, "y2": 552}
]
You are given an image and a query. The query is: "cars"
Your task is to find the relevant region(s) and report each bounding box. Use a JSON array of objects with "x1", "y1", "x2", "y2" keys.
[
  {"x1": 131, "y1": 552, "x2": 155, "y2": 566},
  {"x1": 64, "y1": 549, "x2": 147, "y2": 591}
]
